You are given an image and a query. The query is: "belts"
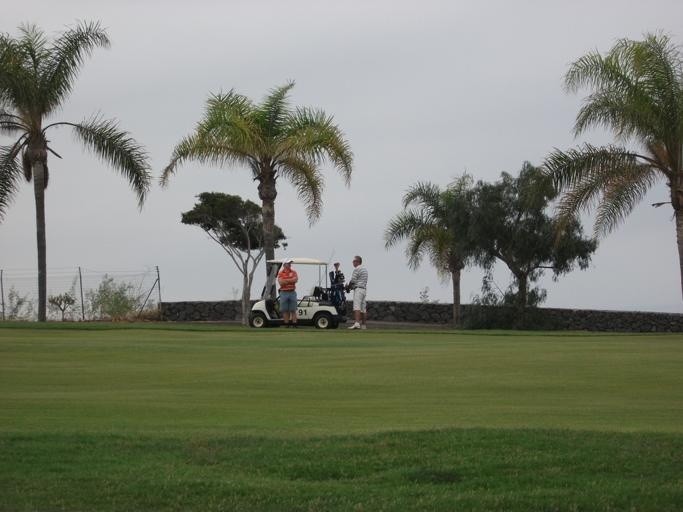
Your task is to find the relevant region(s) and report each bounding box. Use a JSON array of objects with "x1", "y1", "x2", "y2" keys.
[{"x1": 355, "y1": 286, "x2": 365, "y2": 289}]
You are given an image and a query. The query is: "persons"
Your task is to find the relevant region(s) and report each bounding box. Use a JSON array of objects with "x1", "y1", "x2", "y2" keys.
[
  {"x1": 346, "y1": 255, "x2": 368, "y2": 330},
  {"x1": 277, "y1": 259, "x2": 298, "y2": 327}
]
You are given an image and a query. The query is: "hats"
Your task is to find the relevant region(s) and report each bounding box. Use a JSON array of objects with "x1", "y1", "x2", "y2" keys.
[{"x1": 283, "y1": 258, "x2": 294, "y2": 266}]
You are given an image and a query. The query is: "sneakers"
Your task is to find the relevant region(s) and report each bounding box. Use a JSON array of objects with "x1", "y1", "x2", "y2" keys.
[{"x1": 347, "y1": 321, "x2": 367, "y2": 329}]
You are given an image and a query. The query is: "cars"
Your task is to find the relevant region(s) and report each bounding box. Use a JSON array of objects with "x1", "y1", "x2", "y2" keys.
[{"x1": 248, "y1": 259, "x2": 339, "y2": 329}]
